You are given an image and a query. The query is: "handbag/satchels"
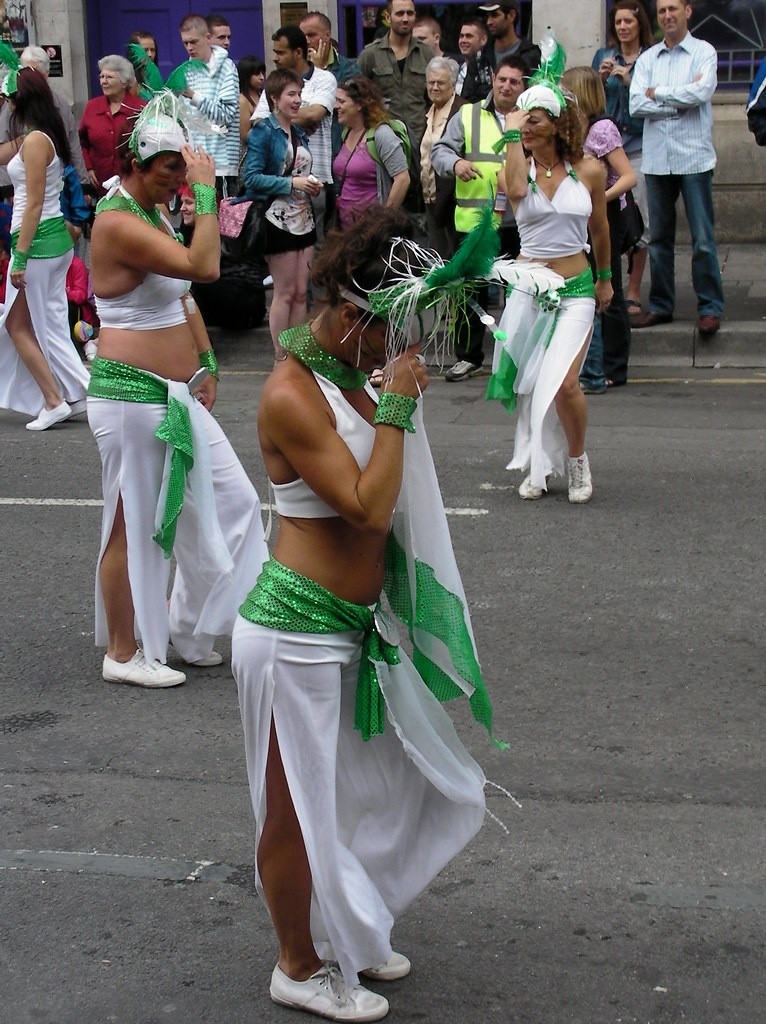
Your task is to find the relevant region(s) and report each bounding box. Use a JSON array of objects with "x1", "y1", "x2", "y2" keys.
[
  {"x1": 218, "y1": 197, "x2": 266, "y2": 257},
  {"x1": 611, "y1": 202, "x2": 643, "y2": 258},
  {"x1": 322, "y1": 205, "x2": 340, "y2": 238}
]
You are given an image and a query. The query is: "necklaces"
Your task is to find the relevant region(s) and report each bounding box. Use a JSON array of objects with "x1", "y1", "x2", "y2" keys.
[
  {"x1": 531, "y1": 154, "x2": 563, "y2": 178},
  {"x1": 624, "y1": 47, "x2": 643, "y2": 66}
]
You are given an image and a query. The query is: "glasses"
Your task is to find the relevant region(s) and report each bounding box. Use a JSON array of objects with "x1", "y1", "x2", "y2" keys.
[{"x1": 345, "y1": 78, "x2": 362, "y2": 98}]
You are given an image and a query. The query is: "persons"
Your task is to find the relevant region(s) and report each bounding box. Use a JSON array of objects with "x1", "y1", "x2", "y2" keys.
[
  {"x1": 0, "y1": 0, "x2": 766, "y2": 433},
  {"x1": 229, "y1": 206, "x2": 492, "y2": 1022},
  {"x1": 86, "y1": 112, "x2": 268, "y2": 688},
  {"x1": 485, "y1": 84, "x2": 614, "y2": 503}
]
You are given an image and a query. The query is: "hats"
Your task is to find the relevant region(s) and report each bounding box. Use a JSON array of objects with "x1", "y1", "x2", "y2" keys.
[{"x1": 479, "y1": 0, "x2": 521, "y2": 13}]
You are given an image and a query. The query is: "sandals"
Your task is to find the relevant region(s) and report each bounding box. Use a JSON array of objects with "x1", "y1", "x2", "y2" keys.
[{"x1": 626, "y1": 299, "x2": 641, "y2": 315}]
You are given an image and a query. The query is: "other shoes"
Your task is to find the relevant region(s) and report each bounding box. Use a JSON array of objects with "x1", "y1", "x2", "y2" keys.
[{"x1": 604, "y1": 378, "x2": 627, "y2": 387}]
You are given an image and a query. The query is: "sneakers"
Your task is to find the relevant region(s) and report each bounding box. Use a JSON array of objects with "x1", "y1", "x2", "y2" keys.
[
  {"x1": 26, "y1": 399, "x2": 71, "y2": 430},
  {"x1": 103, "y1": 643, "x2": 186, "y2": 687},
  {"x1": 58, "y1": 399, "x2": 88, "y2": 423},
  {"x1": 446, "y1": 360, "x2": 483, "y2": 381},
  {"x1": 169, "y1": 635, "x2": 222, "y2": 665},
  {"x1": 270, "y1": 962, "x2": 389, "y2": 1023},
  {"x1": 567, "y1": 452, "x2": 592, "y2": 502},
  {"x1": 579, "y1": 383, "x2": 605, "y2": 394},
  {"x1": 318, "y1": 942, "x2": 410, "y2": 980},
  {"x1": 519, "y1": 473, "x2": 550, "y2": 499}
]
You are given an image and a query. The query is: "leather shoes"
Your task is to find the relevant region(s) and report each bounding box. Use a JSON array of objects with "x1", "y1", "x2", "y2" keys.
[
  {"x1": 630, "y1": 312, "x2": 672, "y2": 326},
  {"x1": 699, "y1": 314, "x2": 720, "y2": 333}
]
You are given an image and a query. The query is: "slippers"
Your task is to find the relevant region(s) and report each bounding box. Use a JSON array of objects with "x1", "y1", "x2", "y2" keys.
[{"x1": 368, "y1": 374, "x2": 383, "y2": 385}]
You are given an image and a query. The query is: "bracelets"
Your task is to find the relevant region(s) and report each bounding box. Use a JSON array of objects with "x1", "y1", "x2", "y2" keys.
[
  {"x1": 199, "y1": 350, "x2": 222, "y2": 381},
  {"x1": 11, "y1": 250, "x2": 28, "y2": 271},
  {"x1": 493, "y1": 130, "x2": 523, "y2": 154},
  {"x1": 596, "y1": 267, "x2": 612, "y2": 280},
  {"x1": 373, "y1": 392, "x2": 417, "y2": 433},
  {"x1": 191, "y1": 182, "x2": 219, "y2": 217}
]
